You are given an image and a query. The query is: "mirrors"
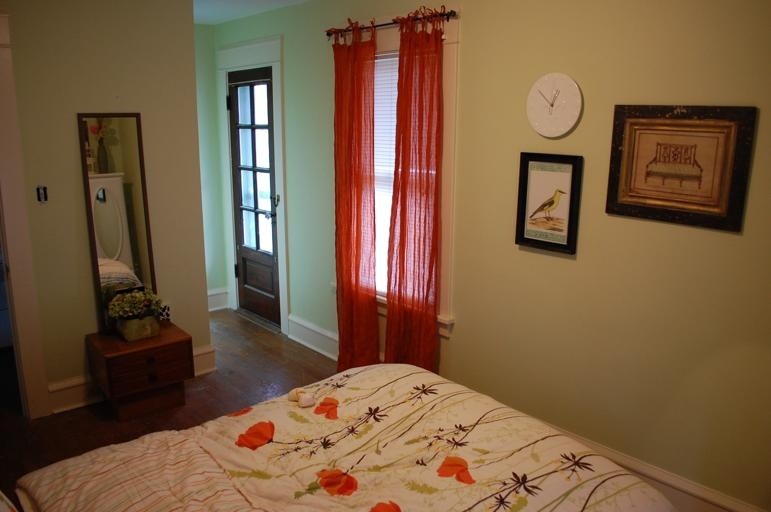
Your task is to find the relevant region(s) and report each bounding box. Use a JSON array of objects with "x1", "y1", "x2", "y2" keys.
[{"x1": 75, "y1": 113, "x2": 157, "y2": 314}]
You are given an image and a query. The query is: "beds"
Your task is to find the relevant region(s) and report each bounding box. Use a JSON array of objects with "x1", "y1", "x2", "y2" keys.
[
  {"x1": 99, "y1": 258, "x2": 143, "y2": 299},
  {"x1": 0, "y1": 363, "x2": 677, "y2": 512}
]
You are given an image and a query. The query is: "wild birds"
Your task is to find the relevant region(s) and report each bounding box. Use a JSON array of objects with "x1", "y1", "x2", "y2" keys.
[{"x1": 529, "y1": 188, "x2": 567, "y2": 221}]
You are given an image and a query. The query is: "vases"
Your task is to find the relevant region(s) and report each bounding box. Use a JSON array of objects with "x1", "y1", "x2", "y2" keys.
[{"x1": 121, "y1": 316, "x2": 163, "y2": 340}]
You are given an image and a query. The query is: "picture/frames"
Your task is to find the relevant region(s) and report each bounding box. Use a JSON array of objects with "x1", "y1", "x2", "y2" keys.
[{"x1": 515, "y1": 106, "x2": 764, "y2": 257}]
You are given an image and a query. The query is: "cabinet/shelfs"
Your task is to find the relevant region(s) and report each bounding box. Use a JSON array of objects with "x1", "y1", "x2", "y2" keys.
[
  {"x1": 88, "y1": 171, "x2": 135, "y2": 272},
  {"x1": 91, "y1": 320, "x2": 195, "y2": 423}
]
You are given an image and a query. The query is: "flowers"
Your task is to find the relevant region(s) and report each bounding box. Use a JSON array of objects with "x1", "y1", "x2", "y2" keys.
[{"x1": 107, "y1": 292, "x2": 172, "y2": 318}]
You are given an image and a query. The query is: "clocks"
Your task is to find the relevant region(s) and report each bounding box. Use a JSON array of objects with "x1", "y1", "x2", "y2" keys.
[{"x1": 525, "y1": 73, "x2": 583, "y2": 139}]
